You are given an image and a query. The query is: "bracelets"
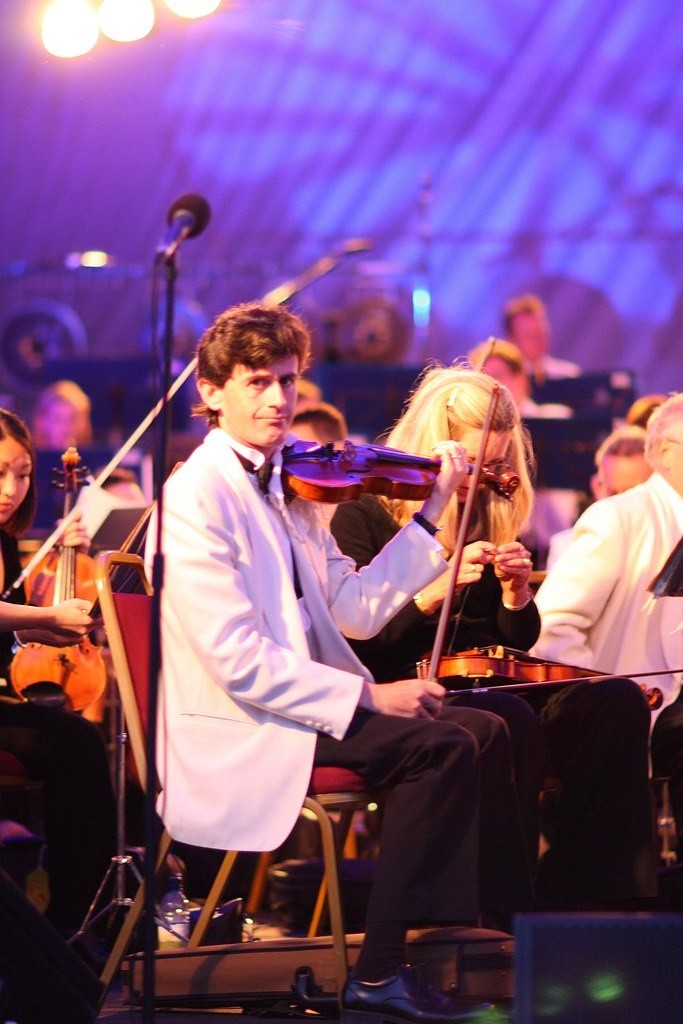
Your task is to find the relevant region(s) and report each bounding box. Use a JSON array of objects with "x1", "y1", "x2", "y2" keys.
[{"x1": 13, "y1": 630, "x2": 26, "y2": 649}]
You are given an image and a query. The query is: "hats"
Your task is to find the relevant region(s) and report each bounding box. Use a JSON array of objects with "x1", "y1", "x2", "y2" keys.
[{"x1": 291, "y1": 402, "x2": 347, "y2": 439}]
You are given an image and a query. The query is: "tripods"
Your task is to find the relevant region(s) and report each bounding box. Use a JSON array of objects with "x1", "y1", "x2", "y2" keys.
[{"x1": 63, "y1": 573, "x2": 189, "y2": 952}]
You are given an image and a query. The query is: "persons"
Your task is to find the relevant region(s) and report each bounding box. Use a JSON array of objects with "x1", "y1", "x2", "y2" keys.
[
  {"x1": 146, "y1": 301, "x2": 524, "y2": 1024},
  {"x1": 0, "y1": 294, "x2": 683, "y2": 908}
]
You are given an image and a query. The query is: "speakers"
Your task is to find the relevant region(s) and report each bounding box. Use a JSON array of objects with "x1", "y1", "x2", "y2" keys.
[
  {"x1": 513, "y1": 909, "x2": 683, "y2": 1024},
  {"x1": 0, "y1": 866, "x2": 106, "y2": 1024}
]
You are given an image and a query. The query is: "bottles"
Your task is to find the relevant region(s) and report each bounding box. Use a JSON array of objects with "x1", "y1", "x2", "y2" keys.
[{"x1": 158, "y1": 871, "x2": 189, "y2": 941}]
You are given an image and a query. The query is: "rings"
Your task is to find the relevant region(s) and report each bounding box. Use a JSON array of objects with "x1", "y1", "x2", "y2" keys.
[{"x1": 471, "y1": 564, "x2": 475, "y2": 573}]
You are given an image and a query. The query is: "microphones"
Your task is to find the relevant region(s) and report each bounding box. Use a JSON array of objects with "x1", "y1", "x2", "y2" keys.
[{"x1": 154, "y1": 194, "x2": 211, "y2": 266}]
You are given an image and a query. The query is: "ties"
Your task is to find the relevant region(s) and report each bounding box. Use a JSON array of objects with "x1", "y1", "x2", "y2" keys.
[{"x1": 233, "y1": 449, "x2": 303, "y2": 599}]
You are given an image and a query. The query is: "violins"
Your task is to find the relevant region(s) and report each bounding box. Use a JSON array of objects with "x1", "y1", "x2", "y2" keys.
[
  {"x1": 281, "y1": 433, "x2": 520, "y2": 506},
  {"x1": 9, "y1": 442, "x2": 106, "y2": 711},
  {"x1": 410, "y1": 644, "x2": 663, "y2": 712}
]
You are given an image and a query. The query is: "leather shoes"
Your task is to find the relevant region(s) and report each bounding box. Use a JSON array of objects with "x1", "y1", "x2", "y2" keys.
[{"x1": 340, "y1": 965, "x2": 495, "y2": 1024}]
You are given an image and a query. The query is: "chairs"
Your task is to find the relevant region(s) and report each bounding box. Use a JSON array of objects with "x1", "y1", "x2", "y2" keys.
[{"x1": 95, "y1": 549, "x2": 392, "y2": 1017}]
